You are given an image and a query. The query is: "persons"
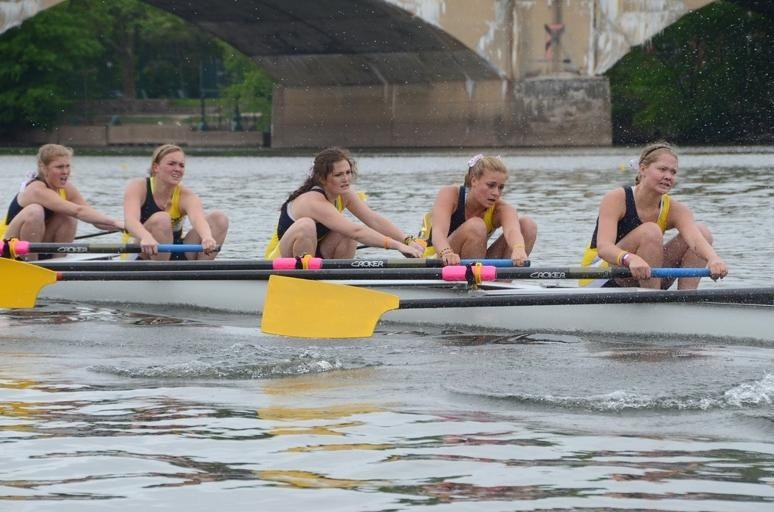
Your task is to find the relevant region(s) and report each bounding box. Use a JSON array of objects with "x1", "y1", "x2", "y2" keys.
[
  {"x1": 0, "y1": 143, "x2": 133, "y2": 262},
  {"x1": 576, "y1": 140, "x2": 729, "y2": 294},
  {"x1": 415, "y1": 149, "x2": 539, "y2": 283},
  {"x1": 123, "y1": 142, "x2": 228, "y2": 264},
  {"x1": 264, "y1": 146, "x2": 427, "y2": 260}
]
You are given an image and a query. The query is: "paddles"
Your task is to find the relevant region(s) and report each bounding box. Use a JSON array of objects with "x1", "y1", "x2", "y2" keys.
[
  {"x1": 0, "y1": 258, "x2": 711, "y2": 314},
  {"x1": 261, "y1": 274, "x2": 774, "y2": 337}
]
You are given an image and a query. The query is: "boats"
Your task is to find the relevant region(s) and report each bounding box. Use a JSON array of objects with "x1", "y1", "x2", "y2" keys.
[{"x1": 26, "y1": 250, "x2": 773, "y2": 348}]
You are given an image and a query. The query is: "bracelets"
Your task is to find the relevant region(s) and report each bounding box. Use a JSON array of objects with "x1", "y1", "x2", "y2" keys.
[
  {"x1": 437, "y1": 246, "x2": 454, "y2": 258},
  {"x1": 383, "y1": 234, "x2": 391, "y2": 250},
  {"x1": 616, "y1": 248, "x2": 629, "y2": 265},
  {"x1": 402, "y1": 233, "x2": 414, "y2": 246}
]
